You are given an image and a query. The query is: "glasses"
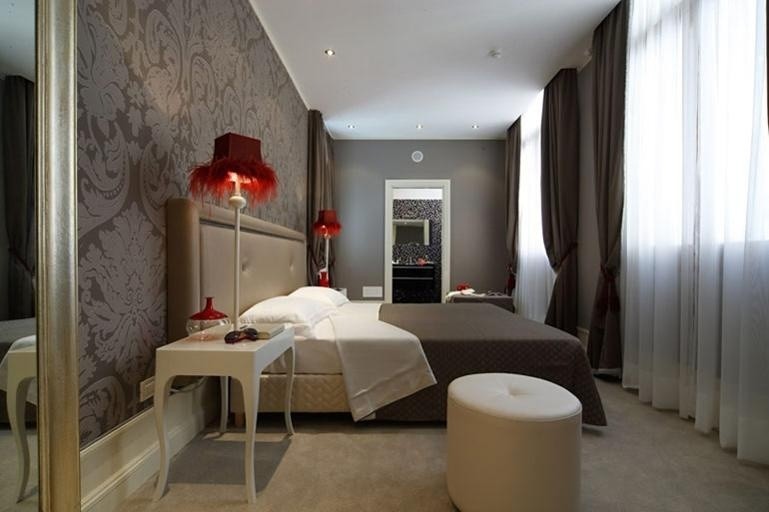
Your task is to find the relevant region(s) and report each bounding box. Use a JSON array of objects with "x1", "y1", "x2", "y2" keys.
[{"x1": 224, "y1": 328, "x2": 258, "y2": 344}]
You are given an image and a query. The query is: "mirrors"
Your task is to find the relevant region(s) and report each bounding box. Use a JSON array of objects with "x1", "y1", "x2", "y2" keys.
[
  {"x1": 391, "y1": 216, "x2": 434, "y2": 246},
  {"x1": 0, "y1": 1, "x2": 82, "y2": 512}
]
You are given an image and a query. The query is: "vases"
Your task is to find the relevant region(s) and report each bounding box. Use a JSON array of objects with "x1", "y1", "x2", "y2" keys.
[{"x1": 184, "y1": 295, "x2": 232, "y2": 341}]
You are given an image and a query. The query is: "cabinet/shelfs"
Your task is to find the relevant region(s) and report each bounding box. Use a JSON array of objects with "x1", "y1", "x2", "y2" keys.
[{"x1": 393, "y1": 265, "x2": 436, "y2": 303}]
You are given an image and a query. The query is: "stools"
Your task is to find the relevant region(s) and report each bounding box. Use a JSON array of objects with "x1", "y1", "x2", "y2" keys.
[{"x1": 446, "y1": 373, "x2": 583, "y2": 509}]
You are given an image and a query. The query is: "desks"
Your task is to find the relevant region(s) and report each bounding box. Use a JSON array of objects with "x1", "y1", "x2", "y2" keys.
[
  {"x1": 149, "y1": 322, "x2": 297, "y2": 505},
  {"x1": 6, "y1": 348, "x2": 38, "y2": 507},
  {"x1": 445, "y1": 291, "x2": 513, "y2": 312}
]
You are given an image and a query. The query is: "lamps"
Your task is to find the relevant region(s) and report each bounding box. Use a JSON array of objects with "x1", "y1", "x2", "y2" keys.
[
  {"x1": 312, "y1": 208, "x2": 342, "y2": 286},
  {"x1": 187, "y1": 132, "x2": 283, "y2": 343}
]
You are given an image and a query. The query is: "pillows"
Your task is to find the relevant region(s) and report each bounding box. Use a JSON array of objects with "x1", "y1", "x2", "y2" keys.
[
  {"x1": 238, "y1": 296, "x2": 333, "y2": 340},
  {"x1": 288, "y1": 284, "x2": 352, "y2": 311}
]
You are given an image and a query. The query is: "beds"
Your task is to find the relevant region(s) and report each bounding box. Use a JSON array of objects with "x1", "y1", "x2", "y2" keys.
[
  {"x1": 166, "y1": 198, "x2": 608, "y2": 426},
  {"x1": 0, "y1": 333, "x2": 38, "y2": 413}
]
